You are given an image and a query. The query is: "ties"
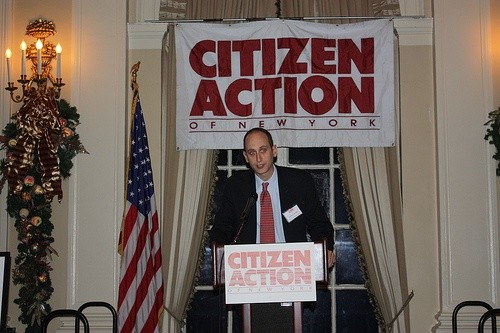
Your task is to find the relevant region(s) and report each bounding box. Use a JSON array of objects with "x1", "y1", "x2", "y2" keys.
[{"x1": 260, "y1": 182, "x2": 275, "y2": 244}]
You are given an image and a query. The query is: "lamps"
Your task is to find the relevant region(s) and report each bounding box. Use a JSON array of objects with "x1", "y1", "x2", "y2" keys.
[{"x1": 5, "y1": 17, "x2": 64, "y2": 100}]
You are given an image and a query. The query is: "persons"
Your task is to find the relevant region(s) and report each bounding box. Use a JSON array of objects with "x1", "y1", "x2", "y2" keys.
[{"x1": 209, "y1": 127, "x2": 337, "y2": 333}]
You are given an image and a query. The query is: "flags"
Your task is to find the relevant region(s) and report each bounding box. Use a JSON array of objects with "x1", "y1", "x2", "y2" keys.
[{"x1": 117, "y1": 80, "x2": 165, "y2": 333}]
